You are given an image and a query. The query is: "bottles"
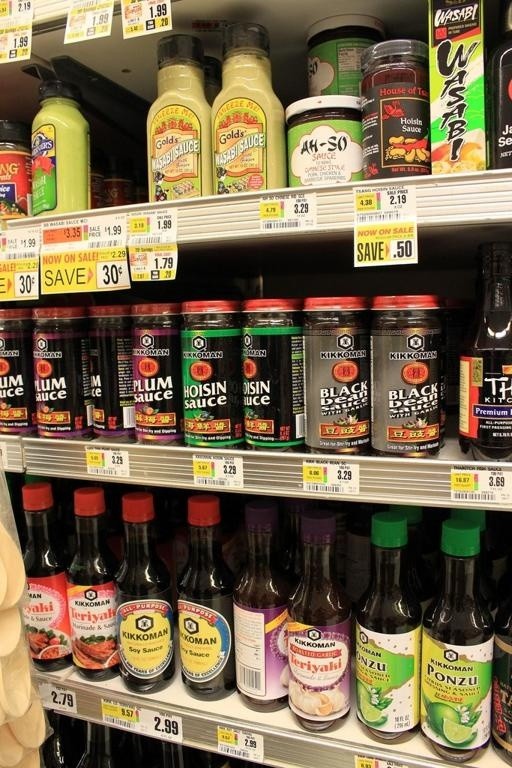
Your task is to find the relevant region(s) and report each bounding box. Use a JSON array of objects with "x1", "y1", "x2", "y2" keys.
[
  {"x1": 16, "y1": 482, "x2": 512, "y2": 768},
  {"x1": 144, "y1": 33, "x2": 213, "y2": 205},
  {"x1": 458, "y1": 263, "x2": 511, "y2": 461},
  {"x1": 490, "y1": 4, "x2": 511, "y2": 169},
  {"x1": 0, "y1": 288, "x2": 445, "y2": 447},
  {"x1": 31, "y1": 81, "x2": 89, "y2": 218},
  {"x1": 210, "y1": 20, "x2": 291, "y2": 199}
]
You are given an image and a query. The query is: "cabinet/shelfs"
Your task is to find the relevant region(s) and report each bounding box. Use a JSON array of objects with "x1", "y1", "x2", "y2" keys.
[{"x1": 0, "y1": 1, "x2": 512, "y2": 768}]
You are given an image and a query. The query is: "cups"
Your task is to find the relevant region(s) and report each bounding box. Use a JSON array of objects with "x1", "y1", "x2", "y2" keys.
[
  {"x1": 0, "y1": 148, "x2": 32, "y2": 219},
  {"x1": 88, "y1": 168, "x2": 130, "y2": 211}
]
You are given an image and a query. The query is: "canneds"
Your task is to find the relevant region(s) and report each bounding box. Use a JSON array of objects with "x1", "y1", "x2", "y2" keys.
[
  {"x1": 283, "y1": 93, "x2": 362, "y2": 188},
  {"x1": 92, "y1": 173, "x2": 102, "y2": 208},
  {"x1": 180, "y1": 300, "x2": 247, "y2": 447},
  {"x1": 102, "y1": 179, "x2": 130, "y2": 206},
  {"x1": 371, "y1": 295, "x2": 442, "y2": 456},
  {"x1": 82, "y1": 304, "x2": 133, "y2": 438},
  {"x1": 130, "y1": 302, "x2": 181, "y2": 446},
  {"x1": 0, "y1": 306, "x2": 33, "y2": 437},
  {"x1": 0, "y1": 140, "x2": 33, "y2": 222},
  {"x1": 305, "y1": 296, "x2": 371, "y2": 456},
  {"x1": 304, "y1": 13, "x2": 378, "y2": 97},
  {"x1": 243, "y1": 299, "x2": 309, "y2": 449},
  {"x1": 32, "y1": 305, "x2": 89, "y2": 439}
]
